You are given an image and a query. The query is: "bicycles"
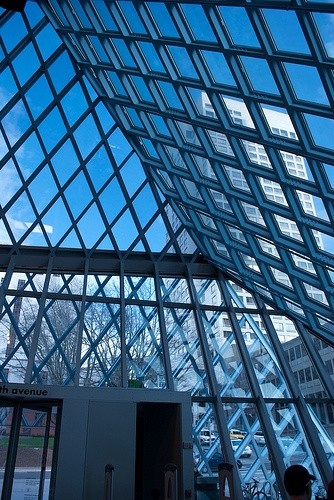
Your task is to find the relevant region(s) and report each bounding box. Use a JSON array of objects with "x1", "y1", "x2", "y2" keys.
[{"x1": 242, "y1": 477, "x2": 268, "y2": 500}]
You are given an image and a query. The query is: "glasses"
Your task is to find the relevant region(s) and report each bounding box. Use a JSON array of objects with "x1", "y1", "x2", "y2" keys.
[{"x1": 305, "y1": 479, "x2": 314, "y2": 486}]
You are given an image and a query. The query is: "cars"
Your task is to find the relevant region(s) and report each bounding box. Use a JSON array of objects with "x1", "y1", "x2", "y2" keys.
[{"x1": 220, "y1": 439, "x2": 252, "y2": 457}]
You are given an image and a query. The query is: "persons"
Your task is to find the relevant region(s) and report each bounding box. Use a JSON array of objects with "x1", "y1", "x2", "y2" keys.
[{"x1": 284, "y1": 465, "x2": 316, "y2": 500}]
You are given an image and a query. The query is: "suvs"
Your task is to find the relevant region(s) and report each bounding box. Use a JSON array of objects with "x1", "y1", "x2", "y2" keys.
[
  {"x1": 230, "y1": 428, "x2": 266, "y2": 444},
  {"x1": 197, "y1": 429, "x2": 216, "y2": 445}
]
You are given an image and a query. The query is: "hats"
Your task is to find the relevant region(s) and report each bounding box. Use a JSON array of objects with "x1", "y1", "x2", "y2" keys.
[{"x1": 284, "y1": 465, "x2": 316, "y2": 485}]
